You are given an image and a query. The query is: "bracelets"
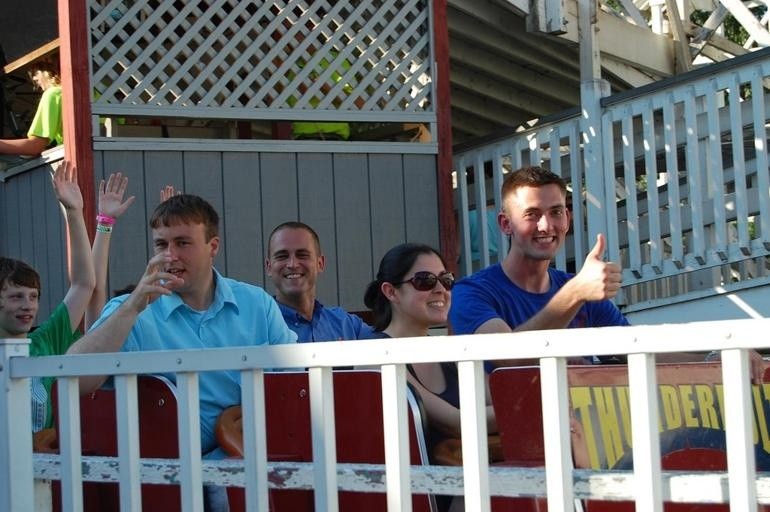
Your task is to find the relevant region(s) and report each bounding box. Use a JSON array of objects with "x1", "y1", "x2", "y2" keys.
[
  {"x1": 94, "y1": 213, "x2": 118, "y2": 223},
  {"x1": 96, "y1": 225, "x2": 116, "y2": 235},
  {"x1": 702, "y1": 349, "x2": 720, "y2": 362}
]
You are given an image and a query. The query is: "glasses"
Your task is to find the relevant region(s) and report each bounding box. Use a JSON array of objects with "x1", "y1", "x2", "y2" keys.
[{"x1": 394, "y1": 271, "x2": 454, "y2": 290}]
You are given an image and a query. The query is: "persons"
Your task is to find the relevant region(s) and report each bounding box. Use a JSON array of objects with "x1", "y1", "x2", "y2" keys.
[
  {"x1": 0, "y1": 54, "x2": 125, "y2": 157},
  {"x1": 365, "y1": 241, "x2": 509, "y2": 443},
  {"x1": 158, "y1": 186, "x2": 185, "y2": 205},
  {"x1": 1, "y1": 159, "x2": 96, "y2": 433},
  {"x1": 263, "y1": 221, "x2": 393, "y2": 342},
  {"x1": 55, "y1": 193, "x2": 302, "y2": 512},
  {"x1": 281, "y1": 32, "x2": 364, "y2": 141},
  {"x1": 446, "y1": 167, "x2": 767, "y2": 406},
  {"x1": 84, "y1": 170, "x2": 134, "y2": 340}
]
